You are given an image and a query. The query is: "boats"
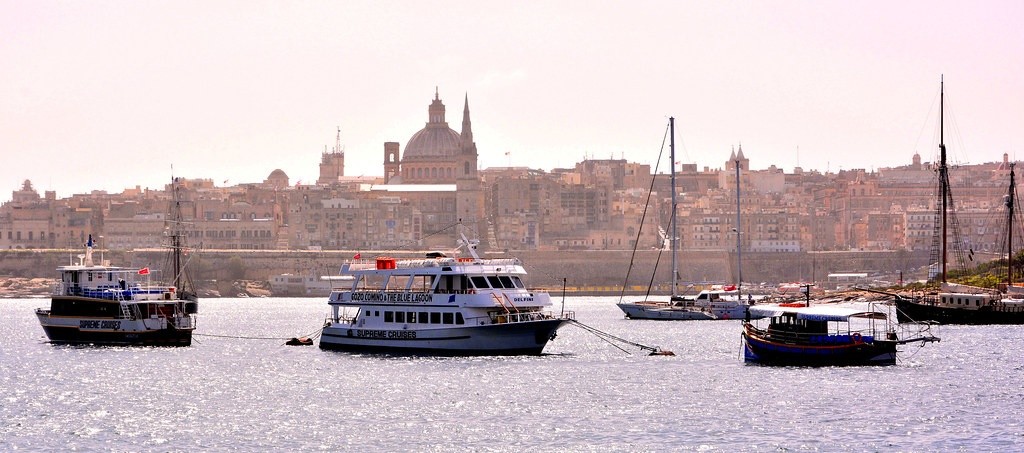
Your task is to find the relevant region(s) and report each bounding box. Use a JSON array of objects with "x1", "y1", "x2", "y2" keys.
[
  {"x1": 737, "y1": 283, "x2": 942, "y2": 368},
  {"x1": 318, "y1": 229, "x2": 574, "y2": 357}
]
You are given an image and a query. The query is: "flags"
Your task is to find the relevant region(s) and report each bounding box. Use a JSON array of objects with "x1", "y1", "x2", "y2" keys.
[
  {"x1": 138, "y1": 267, "x2": 150, "y2": 275},
  {"x1": 724, "y1": 284, "x2": 736, "y2": 291},
  {"x1": 353, "y1": 254, "x2": 360, "y2": 259}
]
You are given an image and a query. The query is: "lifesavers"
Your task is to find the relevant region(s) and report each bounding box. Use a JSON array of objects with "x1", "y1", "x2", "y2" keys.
[
  {"x1": 468, "y1": 289, "x2": 476, "y2": 294},
  {"x1": 852, "y1": 333, "x2": 862, "y2": 344}
]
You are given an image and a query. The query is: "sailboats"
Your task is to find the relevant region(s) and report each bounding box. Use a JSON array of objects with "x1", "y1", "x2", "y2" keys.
[
  {"x1": 968, "y1": 161, "x2": 1024, "y2": 322},
  {"x1": 617, "y1": 115, "x2": 718, "y2": 323},
  {"x1": 860, "y1": 73, "x2": 1024, "y2": 331},
  {"x1": 691, "y1": 159, "x2": 768, "y2": 319},
  {"x1": 35, "y1": 160, "x2": 200, "y2": 349}
]
